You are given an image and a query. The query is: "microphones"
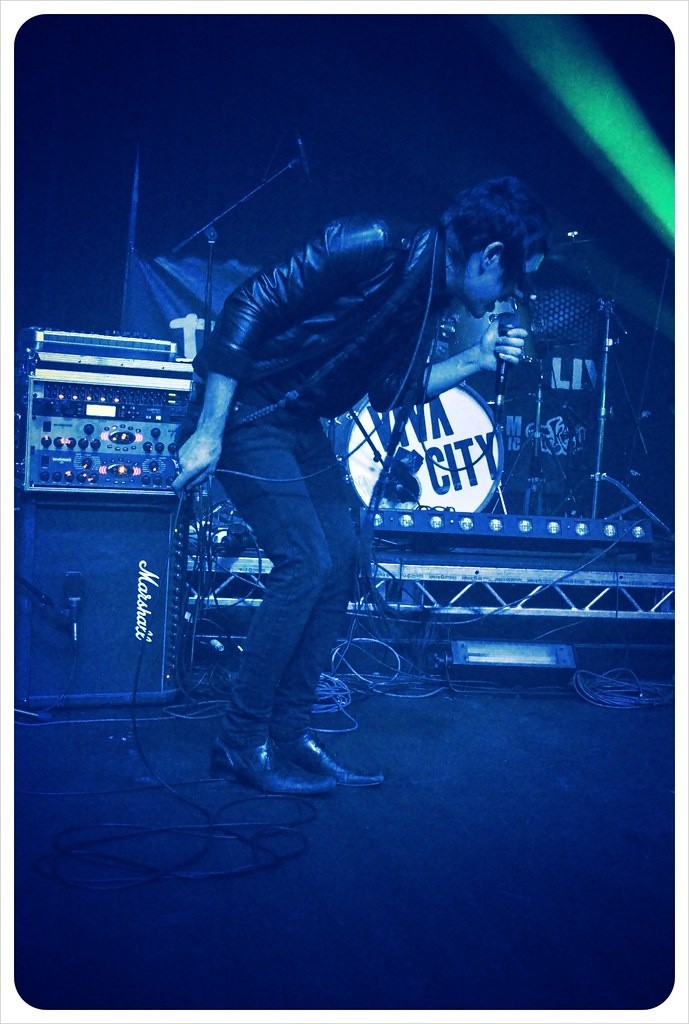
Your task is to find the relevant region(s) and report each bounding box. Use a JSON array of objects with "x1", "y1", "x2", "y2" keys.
[
  {"x1": 390, "y1": 480, "x2": 415, "y2": 498},
  {"x1": 64, "y1": 570, "x2": 86, "y2": 645},
  {"x1": 294, "y1": 126, "x2": 312, "y2": 180},
  {"x1": 494, "y1": 313, "x2": 520, "y2": 424}
]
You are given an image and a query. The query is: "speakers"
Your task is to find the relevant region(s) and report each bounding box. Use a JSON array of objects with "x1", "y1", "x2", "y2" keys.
[{"x1": 14, "y1": 495, "x2": 184, "y2": 708}]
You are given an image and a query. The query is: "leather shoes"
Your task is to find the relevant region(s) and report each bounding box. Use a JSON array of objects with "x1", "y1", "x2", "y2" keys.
[
  {"x1": 276, "y1": 728, "x2": 385, "y2": 788},
  {"x1": 211, "y1": 733, "x2": 336, "y2": 796}
]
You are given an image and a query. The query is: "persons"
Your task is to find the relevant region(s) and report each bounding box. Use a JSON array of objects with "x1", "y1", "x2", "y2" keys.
[{"x1": 172, "y1": 175, "x2": 546, "y2": 796}]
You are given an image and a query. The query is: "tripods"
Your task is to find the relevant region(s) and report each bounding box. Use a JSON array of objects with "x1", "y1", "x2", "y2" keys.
[{"x1": 490, "y1": 244, "x2": 672, "y2": 536}]
